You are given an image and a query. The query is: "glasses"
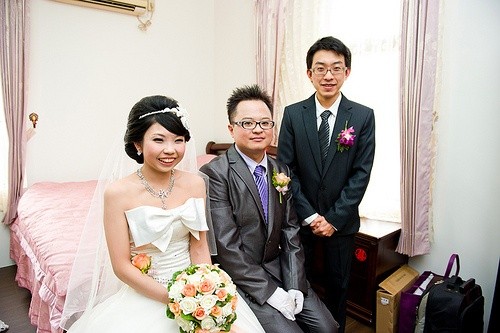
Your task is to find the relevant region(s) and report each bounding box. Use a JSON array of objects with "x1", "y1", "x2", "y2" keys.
[
  {"x1": 230, "y1": 120, "x2": 275, "y2": 130},
  {"x1": 310, "y1": 66, "x2": 347, "y2": 74}
]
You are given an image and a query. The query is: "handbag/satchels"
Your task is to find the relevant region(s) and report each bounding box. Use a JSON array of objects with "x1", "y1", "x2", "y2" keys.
[{"x1": 394, "y1": 254, "x2": 462, "y2": 333}]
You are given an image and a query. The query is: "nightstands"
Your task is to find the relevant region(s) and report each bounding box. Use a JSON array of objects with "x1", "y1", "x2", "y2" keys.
[{"x1": 347, "y1": 217, "x2": 409, "y2": 327}]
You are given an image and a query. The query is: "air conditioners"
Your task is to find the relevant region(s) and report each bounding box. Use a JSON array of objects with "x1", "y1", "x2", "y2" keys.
[{"x1": 43, "y1": 0, "x2": 155, "y2": 31}]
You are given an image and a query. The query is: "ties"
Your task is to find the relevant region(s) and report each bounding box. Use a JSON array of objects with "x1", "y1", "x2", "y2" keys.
[
  {"x1": 319, "y1": 110, "x2": 332, "y2": 173},
  {"x1": 253, "y1": 166, "x2": 270, "y2": 224}
]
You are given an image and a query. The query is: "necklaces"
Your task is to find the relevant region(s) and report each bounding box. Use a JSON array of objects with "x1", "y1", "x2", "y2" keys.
[{"x1": 136, "y1": 168, "x2": 176, "y2": 210}]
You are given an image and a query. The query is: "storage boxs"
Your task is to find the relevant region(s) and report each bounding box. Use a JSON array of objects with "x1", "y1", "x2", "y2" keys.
[{"x1": 377, "y1": 265, "x2": 440, "y2": 333}]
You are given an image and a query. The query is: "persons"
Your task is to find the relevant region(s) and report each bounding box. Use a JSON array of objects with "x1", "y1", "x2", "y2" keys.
[
  {"x1": 58, "y1": 95, "x2": 266, "y2": 333},
  {"x1": 276, "y1": 35, "x2": 376, "y2": 333},
  {"x1": 198, "y1": 84, "x2": 341, "y2": 333}
]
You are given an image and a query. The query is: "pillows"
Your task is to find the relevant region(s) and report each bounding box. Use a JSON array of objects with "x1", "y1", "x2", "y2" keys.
[{"x1": 26, "y1": 128, "x2": 197, "y2": 186}]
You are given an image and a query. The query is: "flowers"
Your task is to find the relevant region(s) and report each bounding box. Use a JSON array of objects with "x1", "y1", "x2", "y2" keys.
[
  {"x1": 130, "y1": 253, "x2": 152, "y2": 275},
  {"x1": 335, "y1": 120, "x2": 356, "y2": 152},
  {"x1": 272, "y1": 168, "x2": 291, "y2": 204},
  {"x1": 166, "y1": 264, "x2": 237, "y2": 333}
]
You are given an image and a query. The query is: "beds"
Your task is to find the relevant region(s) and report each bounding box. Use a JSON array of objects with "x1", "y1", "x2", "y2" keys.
[{"x1": 9, "y1": 183, "x2": 278, "y2": 333}]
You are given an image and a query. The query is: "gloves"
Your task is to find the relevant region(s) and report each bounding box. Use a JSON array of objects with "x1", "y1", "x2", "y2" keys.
[
  {"x1": 287, "y1": 289, "x2": 304, "y2": 315},
  {"x1": 266, "y1": 286, "x2": 297, "y2": 321}
]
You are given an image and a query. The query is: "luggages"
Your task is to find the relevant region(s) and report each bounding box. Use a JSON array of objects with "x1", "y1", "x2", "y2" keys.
[{"x1": 415, "y1": 275, "x2": 486, "y2": 333}]
